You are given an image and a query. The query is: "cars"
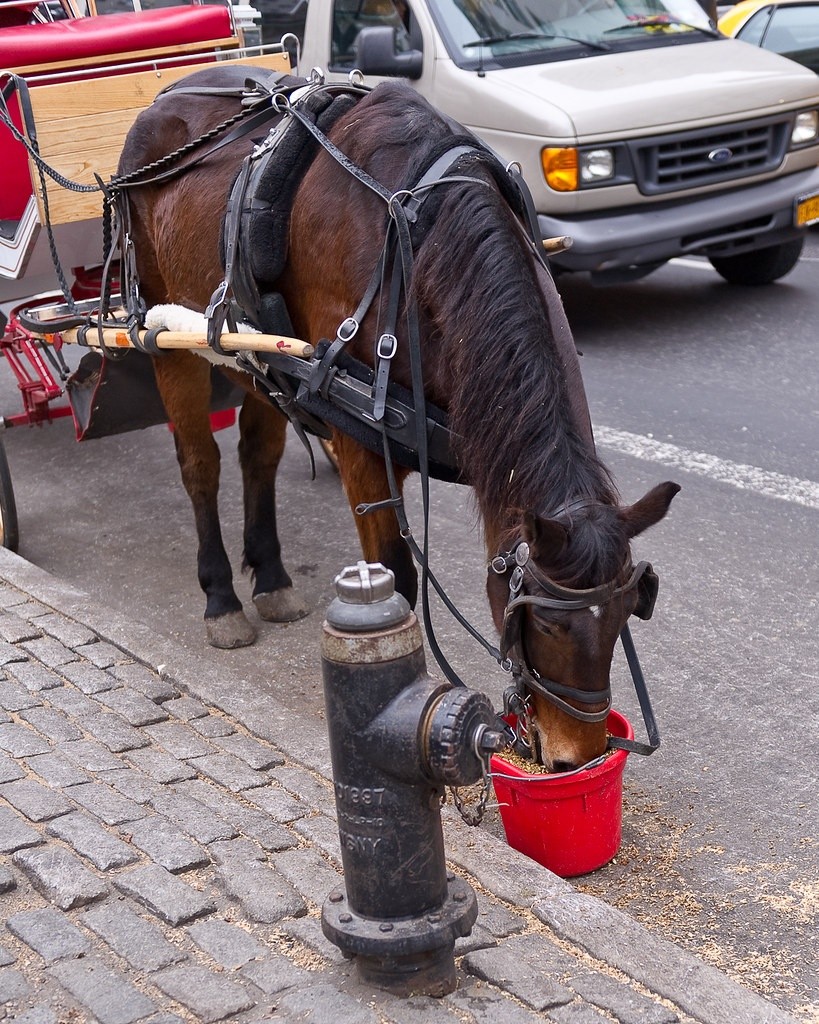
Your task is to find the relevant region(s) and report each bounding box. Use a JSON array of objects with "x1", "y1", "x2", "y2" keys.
[{"x1": 704, "y1": 1, "x2": 819, "y2": 80}]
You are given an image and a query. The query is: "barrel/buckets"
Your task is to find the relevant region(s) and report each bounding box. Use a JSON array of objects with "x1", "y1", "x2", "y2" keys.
[{"x1": 485, "y1": 704, "x2": 634, "y2": 877}]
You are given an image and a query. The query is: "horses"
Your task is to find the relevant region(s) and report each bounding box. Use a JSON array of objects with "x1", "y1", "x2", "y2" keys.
[{"x1": 109, "y1": 61, "x2": 686, "y2": 775}]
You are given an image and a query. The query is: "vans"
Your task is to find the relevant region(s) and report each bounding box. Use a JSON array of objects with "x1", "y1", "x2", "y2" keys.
[{"x1": 66, "y1": 0, "x2": 819, "y2": 301}]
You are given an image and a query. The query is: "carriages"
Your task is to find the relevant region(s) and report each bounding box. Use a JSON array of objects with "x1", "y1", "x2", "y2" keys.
[{"x1": 0, "y1": 1, "x2": 688, "y2": 781}]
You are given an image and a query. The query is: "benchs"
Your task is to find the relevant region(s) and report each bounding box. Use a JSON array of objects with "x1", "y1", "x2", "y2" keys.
[{"x1": 0, "y1": 4, "x2": 235, "y2": 222}]
